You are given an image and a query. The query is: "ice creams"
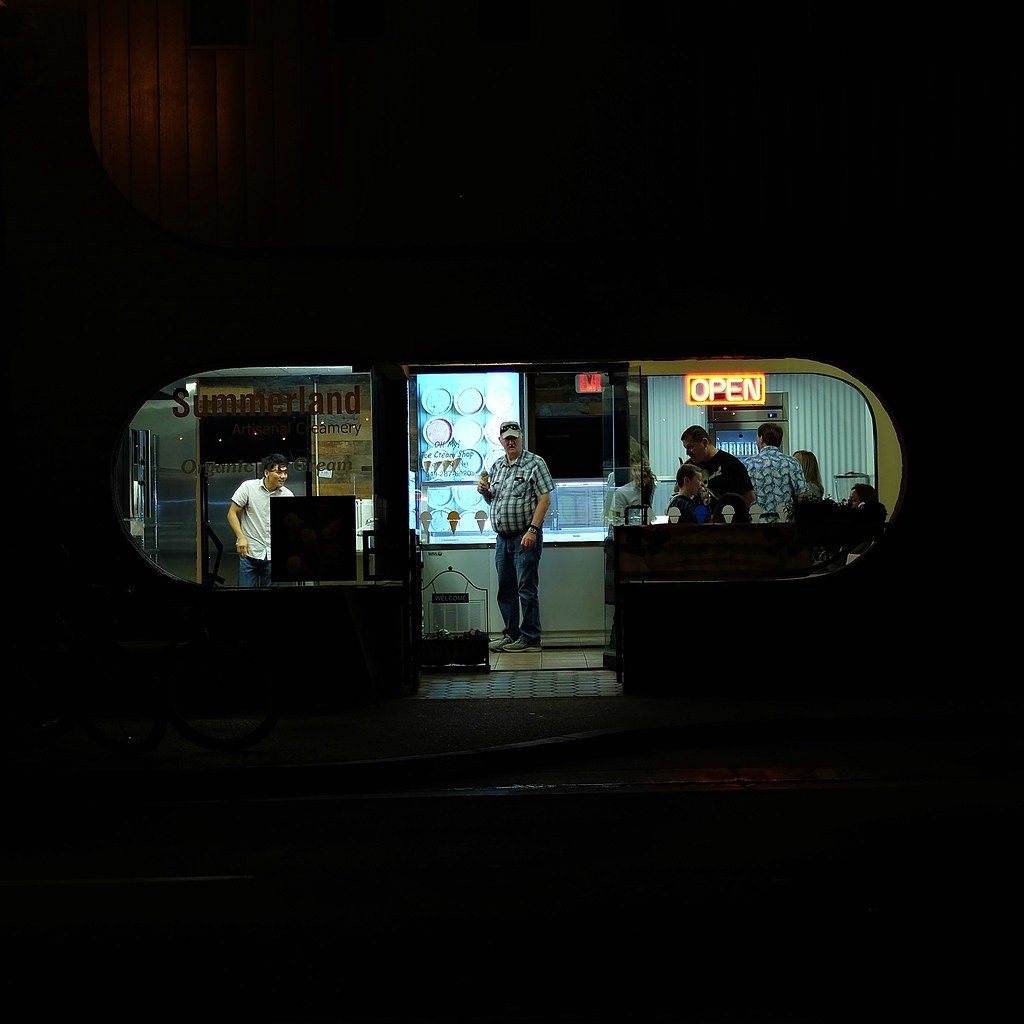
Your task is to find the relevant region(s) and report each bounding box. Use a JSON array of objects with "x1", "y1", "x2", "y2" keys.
[
  {"x1": 423, "y1": 455, "x2": 462, "y2": 474},
  {"x1": 481, "y1": 471, "x2": 490, "y2": 490}
]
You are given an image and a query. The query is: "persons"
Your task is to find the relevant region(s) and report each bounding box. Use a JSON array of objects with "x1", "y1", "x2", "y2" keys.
[
  {"x1": 608, "y1": 441, "x2": 657, "y2": 523},
  {"x1": 667, "y1": 422, "x2": 878, "y2": 523},
  {"x1": 477, "y1": 421, "x2": 555, "y2": 652},
  {"x1": 227, "y1": 454, "x2": 294, "y2": 587}
]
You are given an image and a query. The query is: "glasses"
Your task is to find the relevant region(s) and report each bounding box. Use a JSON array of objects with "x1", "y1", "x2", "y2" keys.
[{"x1": 500, "y1": 424, "x2": 520, "y2": 435}]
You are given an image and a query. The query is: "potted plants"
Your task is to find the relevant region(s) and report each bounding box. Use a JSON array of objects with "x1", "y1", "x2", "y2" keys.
[{"x1": 419, "y1": 625, "x2": 490, "y2": 673}]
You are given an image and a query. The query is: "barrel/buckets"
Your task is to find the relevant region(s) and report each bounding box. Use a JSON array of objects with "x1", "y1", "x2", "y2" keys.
[{"x1": 834, "y1": 471, "x2": 870, "y2": 503}]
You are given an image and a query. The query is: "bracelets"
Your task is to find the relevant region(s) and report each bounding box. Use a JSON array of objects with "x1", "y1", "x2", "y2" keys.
[{"x1": 530, "y1": 525, "x2": 538, "y2": 530}]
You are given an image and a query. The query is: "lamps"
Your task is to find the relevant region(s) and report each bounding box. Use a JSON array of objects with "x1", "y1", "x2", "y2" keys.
[{"x1": 185, "y1": 379, "x2": 197, "y2": 395}]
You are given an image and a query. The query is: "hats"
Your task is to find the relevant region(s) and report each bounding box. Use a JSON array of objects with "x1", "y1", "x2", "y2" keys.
[{"x1": 500, "y1": 422, "x2": 521, "y2": 439}]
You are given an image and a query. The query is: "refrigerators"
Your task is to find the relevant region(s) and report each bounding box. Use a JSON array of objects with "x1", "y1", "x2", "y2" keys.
[{"x1": 703, "y1": 391, "x2": 792, "y2": 470}]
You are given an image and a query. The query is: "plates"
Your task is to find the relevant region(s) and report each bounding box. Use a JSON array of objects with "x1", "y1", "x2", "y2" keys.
[{"x1": 419, "y1": 385, "x2": 513, "y2": 532}]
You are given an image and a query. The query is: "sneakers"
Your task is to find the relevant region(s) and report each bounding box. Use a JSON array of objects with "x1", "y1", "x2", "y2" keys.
[
  {"x1": 502, "y1": 638, "x2": 542, "y2": 653},
  {"x1": 488, "y1": 634, "x2": 513, "y2": 652}
]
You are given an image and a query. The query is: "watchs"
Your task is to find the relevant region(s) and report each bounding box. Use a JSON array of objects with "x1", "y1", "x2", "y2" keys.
[{"x1": 529, "y1": 528, "x2": 536, "y2": 533}]
